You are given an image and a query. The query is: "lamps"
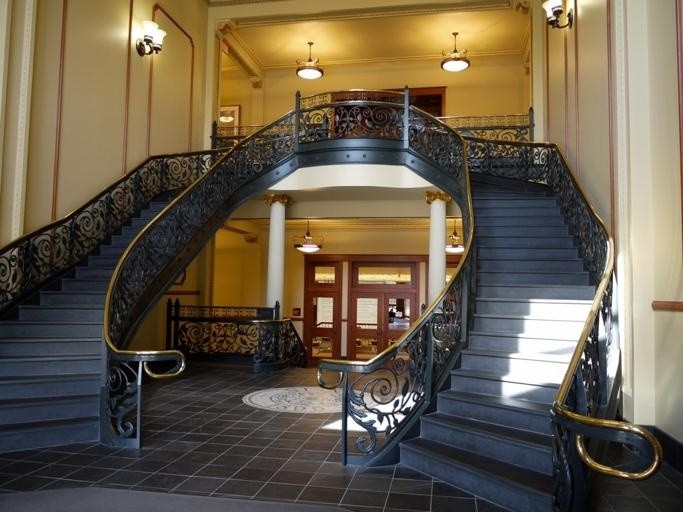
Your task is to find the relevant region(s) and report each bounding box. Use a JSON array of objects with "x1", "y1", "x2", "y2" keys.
[
  {"x1": 294, "y1": 41, "x2": 323, "y2": 79},
  {"x1": 440, "y1": 31, "x2": 470, "y2": 72},
  {"x1": 293, "y1": 221, "x2": 325, "y2": 254},
  {"x1": 136, "y1": 20, "x2": 168, "y2": 56},
  {"x1": 445, "y1": 220, "x2": 466, "y2": 254},
  {"x1": 541, "y1": 0, "x2": 573, "y2": 29}
]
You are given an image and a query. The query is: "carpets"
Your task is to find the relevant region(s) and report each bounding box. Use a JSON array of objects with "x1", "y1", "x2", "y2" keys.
[{"x1": 242, "y1": 386, "x2": 381, "y2": 413}]
